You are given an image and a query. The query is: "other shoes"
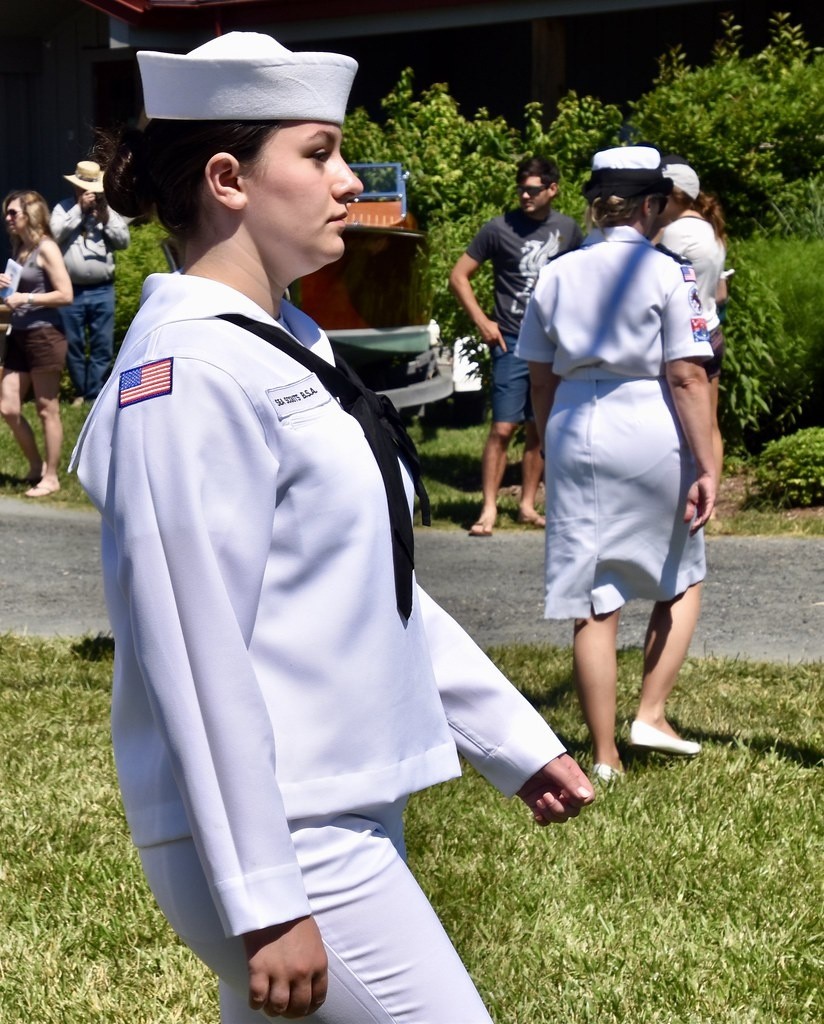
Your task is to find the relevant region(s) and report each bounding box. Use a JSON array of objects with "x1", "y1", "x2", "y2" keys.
[
  {"x1": 629, "y1": 720, "x2": 701, "y2": 754},
  {"x1": 593, "y1": 760, "x2": 625, "y2": 783},
  {"x1": 70, "y1": 397, "x2": 85, "y2": 407}
]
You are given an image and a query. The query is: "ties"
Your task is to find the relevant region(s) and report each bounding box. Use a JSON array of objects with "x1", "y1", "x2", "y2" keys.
[{"x1": 221, "y1": 314, "x2": 431, "y2": 623}]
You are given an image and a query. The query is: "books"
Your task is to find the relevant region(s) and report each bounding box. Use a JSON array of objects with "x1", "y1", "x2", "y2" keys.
[{"x1": 0, "y1": 259, "x2": 23, "y2": 299}]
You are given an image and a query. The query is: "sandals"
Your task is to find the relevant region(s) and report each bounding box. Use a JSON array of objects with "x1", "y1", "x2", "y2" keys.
[{"x1": 25, "y1": 475, "x2": 60, "y2": 497}]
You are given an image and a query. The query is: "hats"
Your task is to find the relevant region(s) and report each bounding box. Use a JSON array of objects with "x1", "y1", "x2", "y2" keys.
[
  {"x1": 582, "y1": 146, "x2": 674, "y2": 200},
  {"x1": 663, "y1": 165, "x2": 700, "y2": 200},
  {"x1": 64, "y1": 160, "x2": 105, "y2": 192},
  {"x1": 136, "y1": 28, "x2": 358, "y2": 122}
]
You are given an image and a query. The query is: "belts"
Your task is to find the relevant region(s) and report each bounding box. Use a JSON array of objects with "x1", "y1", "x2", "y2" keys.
[{"x1": 73, "y1": 281, "x2": 113, "y2": 288}]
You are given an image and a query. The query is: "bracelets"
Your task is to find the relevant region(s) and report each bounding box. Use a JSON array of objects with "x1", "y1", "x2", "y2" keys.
[{"x1": 28, "y1": 293, "x2": 33, "y2": 304}]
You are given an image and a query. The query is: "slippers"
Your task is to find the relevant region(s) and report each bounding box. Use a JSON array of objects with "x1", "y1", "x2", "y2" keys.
[
  {"x1": 517, "y1": 512, "x2": 547, "y2": 528},
  {"x1": 470, "y1": 515, "x2": 496, "y2": 535}
]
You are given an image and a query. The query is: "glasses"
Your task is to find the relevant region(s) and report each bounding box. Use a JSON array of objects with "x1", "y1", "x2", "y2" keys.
[
  {"x1": 515, "y1": 183, "x2": 550, "y2": 196},
  {"x1": 656, "y1": 195, "x2": 668, "y2": 215},
  {"x1": 6, "y1": 209, "x2": 23, "y2": 216}
]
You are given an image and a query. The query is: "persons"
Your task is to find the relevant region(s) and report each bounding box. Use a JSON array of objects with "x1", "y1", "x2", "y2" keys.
[
  {"x1": 0, "y1": 189, "x2": 73, "y2": 497},
  {"x1": 654, "y1": 164, "x2": 727, "y2": 496},
  {"x1": 515, "y1": 143, "x2": 716, "y2": 782},
  {"x1": 48, "y1": 161, "x2": 130, "y2": 408},
  {"x1": 66, "y1": 32, "x2": 595, "y2": 1022},
  {"x1": 451, "y1": 154, "x2": 585, "y2": 536}
]
